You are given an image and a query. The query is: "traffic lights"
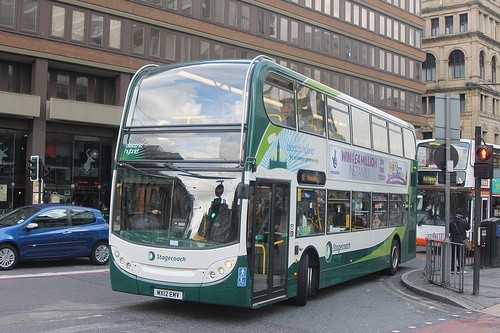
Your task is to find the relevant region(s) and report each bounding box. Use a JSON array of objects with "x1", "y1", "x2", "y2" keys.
[
  {"x1": 28, "y1": 156, "x2": 39, "y2": 181},
  {"x1": 475, "y1": 145, "x2": 494, "y2": 181}
]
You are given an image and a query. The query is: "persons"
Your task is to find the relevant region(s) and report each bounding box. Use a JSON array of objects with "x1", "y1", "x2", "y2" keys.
[
  {"x1": 449, "y1": 211, "x2": 471, "y2": 273},
  {"x1": 267, "y1": 198, "x2": 281, "y2": 255},
  {"x1": 366, "y1": 212, "x2": 380, "y2": 229},
  {"x1": 281, "y1": 105, "x2": 299, "y2": 130},
  {"x1": 296, "y1": 206, "x2": 307, "y2": 236}
]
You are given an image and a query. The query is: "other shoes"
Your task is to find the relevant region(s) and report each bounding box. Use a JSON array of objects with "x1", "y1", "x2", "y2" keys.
[
  {"x1": 457, "y1": 271, "x2": 467, "y2": 274},
  {"x1": 451, "y1": 271, "x2": 454, "y2": 274}
]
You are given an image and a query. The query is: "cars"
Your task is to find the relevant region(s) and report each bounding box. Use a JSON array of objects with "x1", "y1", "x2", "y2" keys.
[{"x1": 0, "y1": 204, "x2": 110, "y2": 271}]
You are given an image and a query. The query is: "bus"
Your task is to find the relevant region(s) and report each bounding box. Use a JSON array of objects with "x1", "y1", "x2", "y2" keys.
[
  {"x1": 415, "y1": 138, "x2": 500, "y2": 253},
  {"x1": 108, "y1": 55, "x2": 418, "y2": 310}
]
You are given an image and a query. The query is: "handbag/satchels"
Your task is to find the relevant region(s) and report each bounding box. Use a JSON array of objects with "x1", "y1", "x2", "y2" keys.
[{"x1": 462, "y1": 239, "x2": 470, "y2": 251}]
